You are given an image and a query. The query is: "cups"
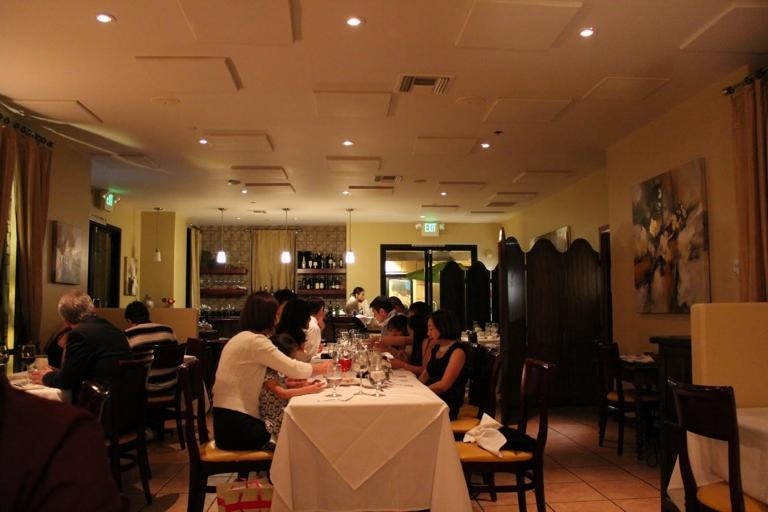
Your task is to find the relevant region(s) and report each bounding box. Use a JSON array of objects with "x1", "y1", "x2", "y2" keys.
[
  {"x1": 0, "y1": 345, "x2": 9, "y2": 364},
  {"x1": 36, "y1": 355, "x2": 52, "y2": 372}
]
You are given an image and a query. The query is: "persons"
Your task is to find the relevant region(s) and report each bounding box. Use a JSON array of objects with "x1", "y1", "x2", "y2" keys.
[
  {"x1": 124, "y1": 302, "x2": 179, "y2": 442},
  {"x1": 0, "y1": 373, "x2": 121, "y2": 512},
  {"x1": 29, "y1": 290, "x2": 130, "y2": 438},
  {"x1": 45, "y1": 325, "x2": 72, "y2": 368}
]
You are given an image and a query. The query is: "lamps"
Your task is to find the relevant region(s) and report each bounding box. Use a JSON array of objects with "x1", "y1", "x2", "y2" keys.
[
  {"x1": 342, "y1": 206, "x2": 356, "y2": 265},
  {"x1": 215, "y1": 206, "x2": 230, "y2": 265},
  {"x1": 150, "y1": 207, "x2": 166, "y2": 265},
  {"x1": 279, "y1": 207, "x2": 294, "y2": 265}
]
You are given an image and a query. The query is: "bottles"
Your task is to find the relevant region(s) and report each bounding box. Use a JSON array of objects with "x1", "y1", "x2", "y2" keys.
[
  {"x1": 324, "y1": 300, "x2": 340, "y2": 317},
  {"x1": 300, "y1": 254, "x2": 344, "y2": 290},
  {"x1": 143, "y1": 293, "x2": 154, "y2": 308}
]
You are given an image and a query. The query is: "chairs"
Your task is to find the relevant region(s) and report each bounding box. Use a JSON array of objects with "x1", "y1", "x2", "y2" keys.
[
  {"x1": 659, "y1": 376, "x2": 768, "y2": 512},
  {"x1": 4, "y1": 317, "x2": 556, "y2": 512},
  {"x1": 592, "y1": 337, "x2": 663, "y2": 455}
]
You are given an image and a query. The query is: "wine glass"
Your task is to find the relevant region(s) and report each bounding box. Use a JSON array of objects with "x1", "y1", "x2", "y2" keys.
[
  {"x1": 22, "y1": 345, "x2": 35, "y2": 373},
  {"x1": 467, "y1": 318, "x2": 498, "y2": 342},
  {"x1": 324, "y1": 327, "x2": 390, "y2": 399}
]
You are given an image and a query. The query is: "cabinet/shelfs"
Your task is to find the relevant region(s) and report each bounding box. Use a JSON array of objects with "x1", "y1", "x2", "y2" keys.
[
  {"x1": 294, "y1": 267, "x2": 347, "y2": 295},
  {"x1": 199, "y1": 264, "x2": 249, "y2": 318},
  {"x1": 648, "y1": 334, "x2": 691, "y2": 383}
]
